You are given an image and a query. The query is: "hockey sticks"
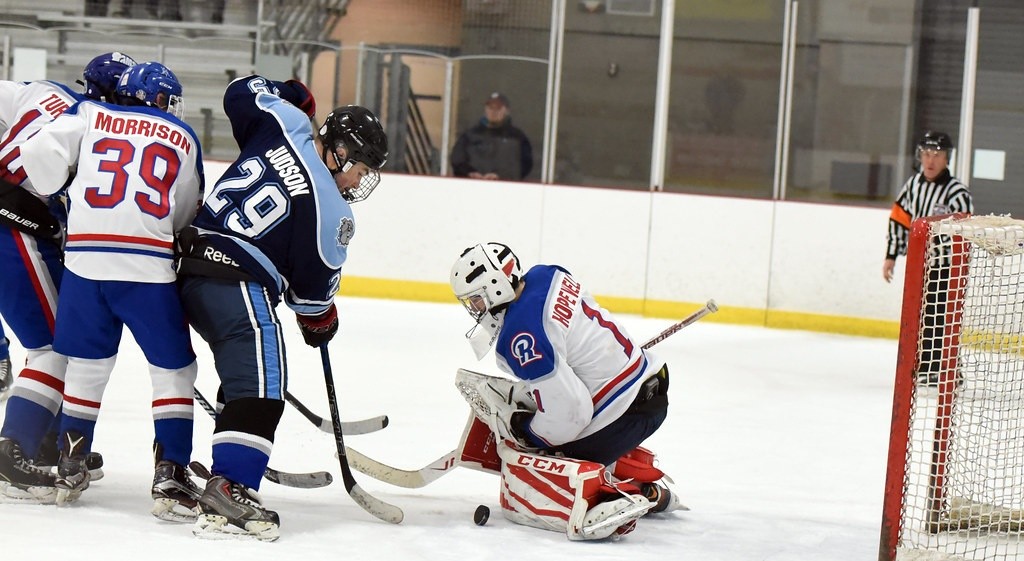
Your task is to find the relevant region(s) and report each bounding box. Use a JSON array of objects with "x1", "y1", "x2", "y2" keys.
[
  {"x1": 333, "y1": 297, "x2": 720, "y2": 488},
  {"x1": 194, "y1": 385, "x2": 334, "y2": 489},
  {"x1": 320, "y1": 343, "x2": 404, "y2": 524},
  {"x1": 284, "y1": 388, "x2": 390, "y2": 436}
]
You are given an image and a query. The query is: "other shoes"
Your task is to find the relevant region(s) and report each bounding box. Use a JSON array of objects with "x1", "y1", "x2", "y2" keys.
[
  {"x1": 583, "y1": 493, "x2": 658, "y2": 539},
  {"x1": 645, "y1": 482, "x2": 691, "y2": 514},
  {"x1": 0, "y1": 356, "x2": 13, "y2": 402}
]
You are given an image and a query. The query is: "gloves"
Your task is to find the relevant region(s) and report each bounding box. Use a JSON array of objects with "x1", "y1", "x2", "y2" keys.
[
  {"x1": 296, "y1": 304, "x2": 339, "y2": 348},
  {"x1": 283, "y1": 79, "x2": 315, "y2": 121}
]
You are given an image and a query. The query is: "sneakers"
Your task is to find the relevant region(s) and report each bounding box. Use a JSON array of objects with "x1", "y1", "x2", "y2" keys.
[
  {"x1": 150, "y1": 440, "x2": 204, "y2": 524},
  {"x1": 0, "y1": 437, "x2": 58, "y2": 505},
  {"x1": 190, "y1": 461, "x2": 281, "y2": 543},
  {"x1": 55, "y1": 431, "x2": 92, "y2": 507},
  {"x1": 28, "y1": 432, "x2": 104, "y2": 481}
]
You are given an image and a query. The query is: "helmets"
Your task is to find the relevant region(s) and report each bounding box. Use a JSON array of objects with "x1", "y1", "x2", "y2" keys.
[
  {"x1": 914, "y1": 131, "x2": 954, "y2": 164},
  {"x1": 319, "y1": 104, "x2": 387, "y2": 204},
  {"x1": 111, "y1": 62, "x2": 185, "y2": 123},
  {"x1": 75, "y1": 52, "x2": 138, "y2": 103},
  {"x1": 450, "y1": 242, "x2": 524, "y2": 362}
]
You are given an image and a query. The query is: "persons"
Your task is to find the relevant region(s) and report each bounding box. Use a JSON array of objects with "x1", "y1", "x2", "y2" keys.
[
  {"x1": 172, "y1": 74, "x2": 389, "y2": 542},
  {"x1": 0, "y1": 52, "x2": 139, "y2": 505},
  {"x1": 883, "y1": 131, "x2": 973, "y2": 387},
  {"x1": 450, "y1": 241, "x2": 690, "y2": 541},
  {"x1": 451, "y1": 92, "x2": 534, "y2": 182},
  {"x1": 19, "y1": 61, "x2": 206, "y2": 523}
]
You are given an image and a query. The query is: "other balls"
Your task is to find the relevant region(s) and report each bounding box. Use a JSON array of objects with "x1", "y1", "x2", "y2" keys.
[{"x1": 473, "y1": 505, "x2": 491, "y2": 527}]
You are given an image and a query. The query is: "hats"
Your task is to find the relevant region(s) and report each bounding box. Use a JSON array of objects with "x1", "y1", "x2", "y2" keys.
[{"x1": 487, "y1": 92, "x2": 510, "y2": 108}]
